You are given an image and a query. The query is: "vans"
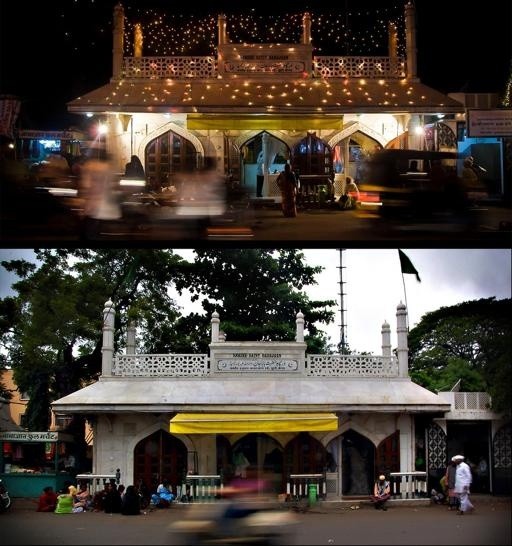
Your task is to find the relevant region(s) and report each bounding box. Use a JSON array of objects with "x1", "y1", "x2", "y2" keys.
[{"x1": 347, "y1": 149, "x2": 512, "y2": 233}]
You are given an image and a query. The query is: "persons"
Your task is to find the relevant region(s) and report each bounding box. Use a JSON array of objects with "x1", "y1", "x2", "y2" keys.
[
  {"x1": 276, "y1": 163, "x2": 299, "y2": 217},
  {"x1": 451, "y1": 455, "x2": 477, "y2": 516},
  {"x1": 255, "y1": 149, "x2": 267, "y2": 198},
  {"x1": 316, "y1": 178, "x2": 338, "y2": 210},
  {"x1": 66, "y1": 143, "x2": 160, "y2": 248},
  {"x1": 342, "y1": 176, "x2": 360, "y2": 211},
  {"x1": 372, "y1": 475, "x2": 392, "y2": 513},
  {"x1": 431, "y1": 455, "x2": 488, "y2": 511},
  {"x1": 465, "y1": 156, "x2": 486, "y2": 187},
  {"x1": 36, "y1": 469, "x2": 199, "y2": 514},
  {"x1": 462, "y1": 159, "x2": 478, "y2": 188}
]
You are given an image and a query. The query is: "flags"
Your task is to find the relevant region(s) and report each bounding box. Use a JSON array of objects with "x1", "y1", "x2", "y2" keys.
[{"x1": 398, "y1": 248, "x2": 421, "y2": 282}]
